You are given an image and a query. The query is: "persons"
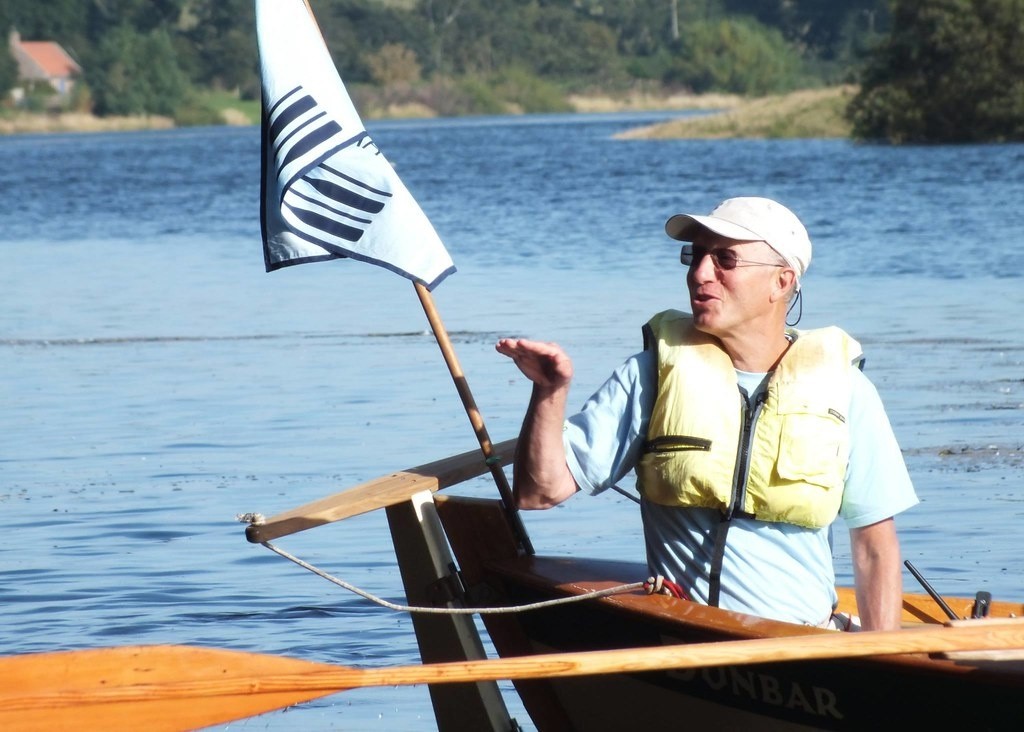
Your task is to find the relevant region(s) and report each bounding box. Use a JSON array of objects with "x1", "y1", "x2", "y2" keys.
[{"x1": 494, "y1": 196, "x2": 920, "y2": 632}]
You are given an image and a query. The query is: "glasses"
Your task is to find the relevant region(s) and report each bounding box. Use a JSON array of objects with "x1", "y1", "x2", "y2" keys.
[{"x1": 681, "y1": 244, "x2": 784, "y2": 270}]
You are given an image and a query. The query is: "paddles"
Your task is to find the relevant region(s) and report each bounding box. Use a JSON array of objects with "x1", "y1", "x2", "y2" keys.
[{"x1": 0, "y1": 617, "x2": 1024, "y2": 731}]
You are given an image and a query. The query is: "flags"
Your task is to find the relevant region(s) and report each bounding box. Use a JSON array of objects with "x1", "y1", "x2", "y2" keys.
[{"x1": 254, "y1": 0, "x2": 457, "y2": 293}]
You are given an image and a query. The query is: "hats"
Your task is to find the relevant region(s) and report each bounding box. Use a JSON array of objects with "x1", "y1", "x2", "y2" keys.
[{"x1": 665, "y1": 196, "x2": 812, "y2": 295}]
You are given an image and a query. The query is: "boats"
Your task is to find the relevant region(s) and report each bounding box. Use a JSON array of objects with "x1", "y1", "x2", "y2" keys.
[{"x1": 243, "y1": 434, "x2": 1024, "y2": 732}]
